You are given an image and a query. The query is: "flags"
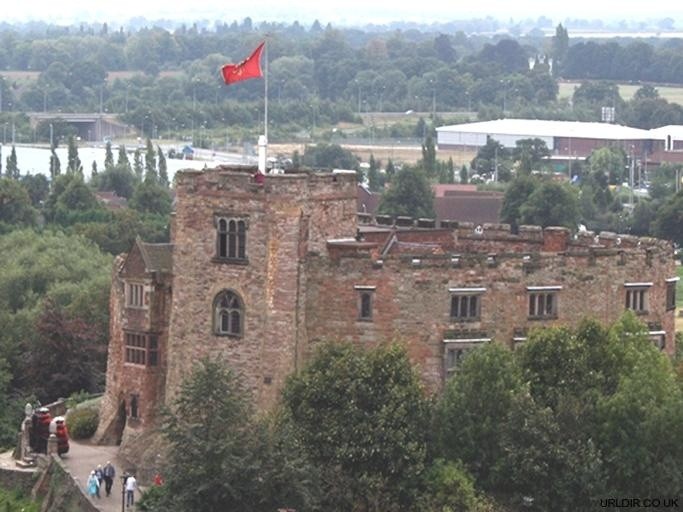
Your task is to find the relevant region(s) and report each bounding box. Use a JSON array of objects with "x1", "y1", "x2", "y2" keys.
[{"x1": 221, "y1": 39, "x2": 265, "y2": 86}]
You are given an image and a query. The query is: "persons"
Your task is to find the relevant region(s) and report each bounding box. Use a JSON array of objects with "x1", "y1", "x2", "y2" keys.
[
  {"x1": 95, "y1": 464, "x2": 103, "y2": 486},
  {"x1": 87, "y1": 470, "x2": 100, "y2": 500},
  {"x1": 125, "y1": 474, "x2": 136, "y2": 507},
  {"x1": 104, "y1": 461, "x2": 115, "y2": 497}
]
